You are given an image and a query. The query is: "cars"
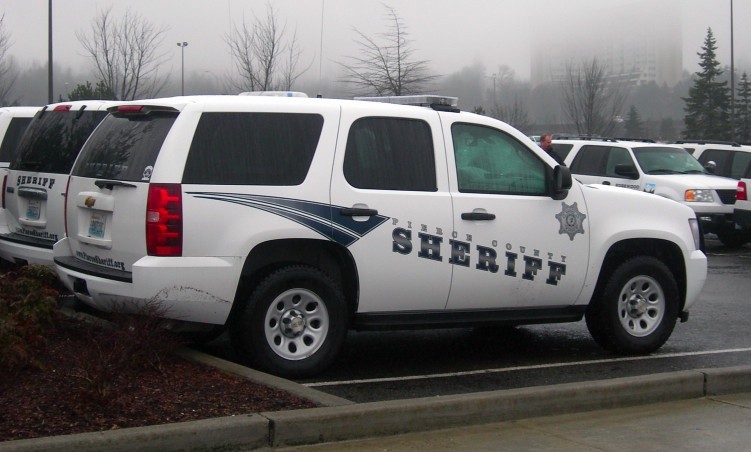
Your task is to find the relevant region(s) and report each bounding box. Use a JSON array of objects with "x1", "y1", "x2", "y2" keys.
[
  {"x1": 673, "y1": 140, "x2": 751, "y2": 247},
  {"x1": 0, "y1": 93, "x2": 133, "y2": 283}
]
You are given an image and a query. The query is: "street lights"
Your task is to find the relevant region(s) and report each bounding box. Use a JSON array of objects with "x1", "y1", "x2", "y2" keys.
[{"x1": 175, "y1": 40, "x2": 194, "y2": 98}]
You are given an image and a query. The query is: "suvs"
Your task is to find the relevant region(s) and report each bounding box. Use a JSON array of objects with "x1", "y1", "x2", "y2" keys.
[
  {"x1": 48, "y1": 91, "x2": 708, "y2": 385},
  {"x1": 526, "y1": 131, "x2": 739, "y2": 242}
]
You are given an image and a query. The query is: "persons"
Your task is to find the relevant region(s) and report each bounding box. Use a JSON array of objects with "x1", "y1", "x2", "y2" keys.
[{"x1": 531, "y1": 135, "x2": 566, "y2": 175}]
